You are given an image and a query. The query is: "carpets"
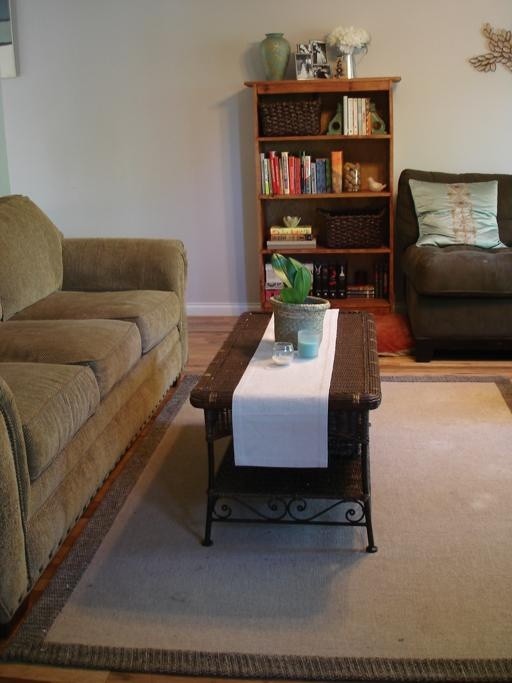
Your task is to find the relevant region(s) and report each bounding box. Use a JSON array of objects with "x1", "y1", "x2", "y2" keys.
[{"x1": 0, "y1": 373, "x2": 512, "y2": 683}]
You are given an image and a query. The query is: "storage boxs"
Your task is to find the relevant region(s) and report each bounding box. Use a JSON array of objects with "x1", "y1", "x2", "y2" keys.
[{"x1": 318, "y1": 204, "x2": 386, "y2": 248}]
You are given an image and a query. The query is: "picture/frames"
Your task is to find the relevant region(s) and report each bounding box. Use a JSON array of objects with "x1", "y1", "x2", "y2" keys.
[{"x1": 294, "y1": 39, "x2": 333, "y2": 80}]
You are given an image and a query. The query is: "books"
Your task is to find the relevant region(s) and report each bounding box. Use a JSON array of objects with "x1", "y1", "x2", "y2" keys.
[
  {"x1": 340, "y1": 92, "x2": 372, "y2": 138},
  {"x1": 260, "y1": 150, "x2": 391, "y2": 303}
]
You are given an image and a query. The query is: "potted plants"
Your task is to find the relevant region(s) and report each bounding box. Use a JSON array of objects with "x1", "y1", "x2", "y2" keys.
[{"x1": 269, "y1": 252, "x2": 331, "y2": 350}]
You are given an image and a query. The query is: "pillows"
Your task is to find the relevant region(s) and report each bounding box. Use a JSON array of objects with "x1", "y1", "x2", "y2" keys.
[{"x1": 408, "y1": 179, "x2": 509, "y2": 249}]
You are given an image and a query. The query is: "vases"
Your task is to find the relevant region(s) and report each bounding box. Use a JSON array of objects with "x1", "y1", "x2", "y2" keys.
[{"x1": 341, "y1": 55, "x2": 355, "y2": 79}]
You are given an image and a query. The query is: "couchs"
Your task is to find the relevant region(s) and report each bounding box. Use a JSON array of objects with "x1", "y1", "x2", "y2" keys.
[{"x1": 0, "y1": 194, "x2": 189, "y2": 629}]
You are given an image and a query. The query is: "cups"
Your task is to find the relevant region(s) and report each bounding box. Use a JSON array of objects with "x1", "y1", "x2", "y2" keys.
[{"x1": 273, "y1": 329, "x2": 318, "y2": 366}]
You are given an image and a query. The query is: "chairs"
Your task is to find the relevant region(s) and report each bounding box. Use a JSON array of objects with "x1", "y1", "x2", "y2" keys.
[{"x1": 396, "y1": 167, "x2": 512, "y2": 360}]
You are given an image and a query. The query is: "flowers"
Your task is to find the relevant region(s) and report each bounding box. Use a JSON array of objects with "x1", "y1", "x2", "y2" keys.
[{"x1": 327, "y1": 25, "x2": 371, "y2": 55}]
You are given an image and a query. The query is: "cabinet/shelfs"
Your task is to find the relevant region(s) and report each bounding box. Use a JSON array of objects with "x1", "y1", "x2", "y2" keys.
[{"x1": 244, "y1": 76, "x2": 402, "y2": 327}]
